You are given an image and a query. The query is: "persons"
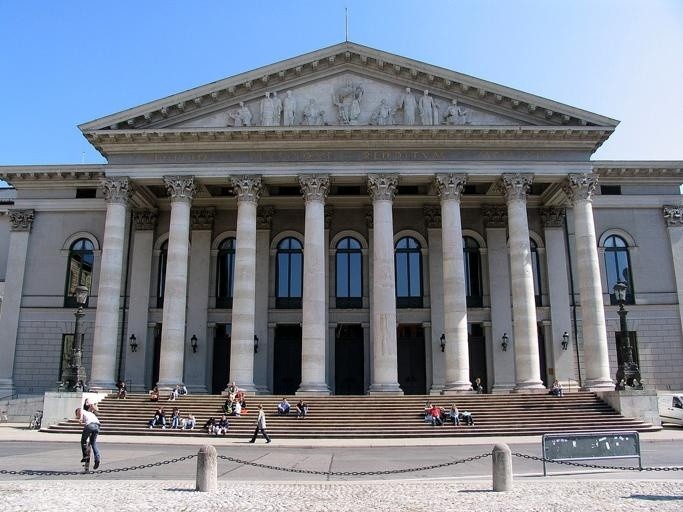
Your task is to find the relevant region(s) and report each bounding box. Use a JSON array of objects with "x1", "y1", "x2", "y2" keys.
[
  {"x1": 551, "y1": 380, "x2": 559, "y2": 396},
  {"x1": 471, "y1": 377, "x2": 482, "y2": 394},
  {"x1": 247, "y1": 403, "x2": 270, "y2": 443},
  {"x1": 295, "y1": 399, "x2": 308, "y2": 418},
  {"x1": 149, "y1": 386, "x2": 159, "y2": 401},
  {"x1": 147, "y1": 405, "x2": 167, "y2": 429},
  {"x1": 228, "y1": 84, "x2": 473, "y2": 128},
  {"x1": 167, "y1": 383, "x2": 187, "y2": 401},
  {"x1": 93, "y1": 403, "x2": 98, "y2": 411},
  {"x1": 114, "y1": 380, "x2": 126, "y2": 399},
  {"x1": 203, "y1": 381, "x2": 245, "y2": 436},
  {"x1": 168, "y1": 407, "x2": 180, "y2": 430},
  {"x1": 83, "y1": 398, "x2": 91, "y2": 410},
  {"x1": 73, "y1": 408, "x2": 101, "y2": 470},
  {"x1": 554, "y1": 379, "x2": 564, "y2": 397},
  {"x1": 181, "y1": 413, "x2": 196, "y2": 431},
  {"x1": 277, "y1": 398, "x2": 290, "y2": 414},
  {"x1": 86, "y1": 404, "x2": 97, "y2": 416},
  {"x1": 421, "y1": 400, "x2": 474, "y2": 427}
]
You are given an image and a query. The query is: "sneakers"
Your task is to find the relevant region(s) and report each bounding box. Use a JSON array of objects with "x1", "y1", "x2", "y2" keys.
[
  {"x1": 93, "y1": 460, "x2": 100, "y2": 469},
  {"x1": 149, "y1": 425, "x2": 153, "y2": 429},
  {"x1": 161, "y1": 425, "x2": 166, "y2": 430},
  {"x1": 81, "y1": 458, "x2": 90, "y2": 463}
]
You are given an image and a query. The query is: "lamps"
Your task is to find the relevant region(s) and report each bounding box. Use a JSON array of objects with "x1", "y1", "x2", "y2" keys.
[
  {"x1": 438, "y1": 333, "x2": 446, "y2": 353},
  {"x1": 500, "y1": 332, "x2": 509, "y2": 353},
  {"x1": 188, "y1": 331, "x2": 199, "y2": 354},
  {"x1": 559, "y1": 330, "x2": 570, "y2": 352},
  {"x1": 127, "y1": 333, "x2": 139, "y2": 352},
  {"x1": 253, "y1": 335, "x2": 258, "y2": 354}
]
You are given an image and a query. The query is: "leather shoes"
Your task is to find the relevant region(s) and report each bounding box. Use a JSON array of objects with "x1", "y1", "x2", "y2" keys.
[
  {"x1": 265, "y1": 439, "x2": 271, "y2": 443},
  {"x1": 249, "y1": 440, "x2": 254, "y2": 443}
]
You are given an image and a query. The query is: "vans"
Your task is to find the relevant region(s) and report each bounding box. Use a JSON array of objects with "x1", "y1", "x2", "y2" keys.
[{"x1": 656, "y1": 390, "x2": 683, "y2": 428}]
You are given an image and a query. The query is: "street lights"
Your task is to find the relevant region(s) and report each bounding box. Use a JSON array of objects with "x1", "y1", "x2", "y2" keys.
[
  {"x1": 68, "y1": 280, "x2": 89, "y2": 366},
  {"x1": 610, "y1": 279, "x2": 636, "y2": 362}
]
